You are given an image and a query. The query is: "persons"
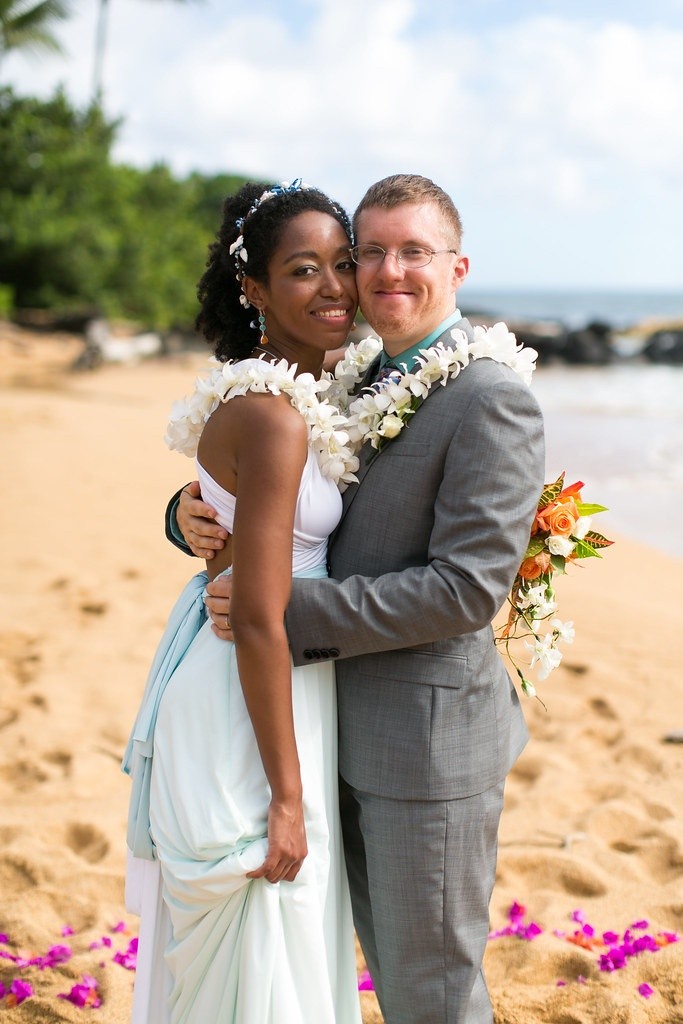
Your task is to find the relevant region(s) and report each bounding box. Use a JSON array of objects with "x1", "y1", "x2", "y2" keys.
[
  {"x1": 130, "y1": 179, "x2": 362, "y2": 1024},
  {"x1": 160, "y1": 170, "x2": 549, "y2": 1023}
]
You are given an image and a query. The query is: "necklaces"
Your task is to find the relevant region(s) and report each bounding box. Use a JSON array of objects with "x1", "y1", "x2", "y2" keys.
[
  {"x1": 152, "y1": 349, "x2": 363, "y2": 495},
  {"x1": 254, "y1": 347, "x2": 281, "y2": 362},
  {"x1": 316, "y1": 318, "x2": 540, "y2": 456}
]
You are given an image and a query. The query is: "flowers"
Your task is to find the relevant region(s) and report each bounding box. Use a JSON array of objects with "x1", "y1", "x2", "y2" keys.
[
  {"x1": 483, "y1": 463, "x2": 616, "y2": 714},
  {"x1": 377, "y1": 413, "x2": 405, "y2": 452}
]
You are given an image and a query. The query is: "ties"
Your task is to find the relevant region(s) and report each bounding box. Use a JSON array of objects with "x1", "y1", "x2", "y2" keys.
[{"x1": 372, "y1": 367, "x2": 402, "y2": 398}]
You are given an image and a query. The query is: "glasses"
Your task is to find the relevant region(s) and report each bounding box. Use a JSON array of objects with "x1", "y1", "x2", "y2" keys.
[{"x1": 348, "y1": 244, "x2": 457, "y2": 268}]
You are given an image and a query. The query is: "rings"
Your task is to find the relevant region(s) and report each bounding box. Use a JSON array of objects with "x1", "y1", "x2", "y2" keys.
[{"x1": 225, "y1": 615, "x2": 232, "y2": 631}]
